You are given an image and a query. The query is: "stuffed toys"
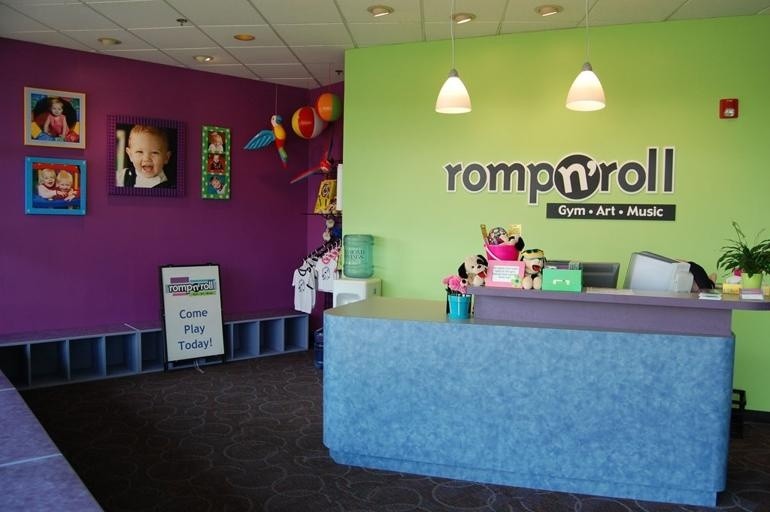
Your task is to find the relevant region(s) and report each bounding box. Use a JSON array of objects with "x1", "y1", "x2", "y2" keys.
[
  {"x1": 519, "y1": 247, "x2": 547, "y2": 290},
  {"x1": 457, "y1": 254, "x2": 489, "y2": 288},
  {"x1": 442, "y1": 274, "x2": 467, "y2": 295}
]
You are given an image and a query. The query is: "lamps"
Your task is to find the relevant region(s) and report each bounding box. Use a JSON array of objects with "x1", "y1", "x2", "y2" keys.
[
  {"x1": 433, "y1": 0, "x2": 473, "y2": 115},
  {"x1": 535, "y1": 4, "x2": 564, "y2": 18},
  {"x1": 565, "y1": 0, "x2": 607, "y2": 112},
  {"x1": 448, "y1": 13, "x2": 476, "y2": 24},
  {"x1": 99, "y1": 38, "x2": 121, "y2": 48},
  {"x1": 193, "y1": 54, "x2": 213, "y2": 65},
  {"x1": 367, "y1": 5, "x2": 394, "y2": 19}
]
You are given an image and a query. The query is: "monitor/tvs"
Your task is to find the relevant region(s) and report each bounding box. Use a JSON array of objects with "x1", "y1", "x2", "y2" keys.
[
  {"x1": 623, "y1": 250, "x2": 694, "y2": 292},
  {"x1": 547, "y1": 260, "x2": 620, "y2": 289}
]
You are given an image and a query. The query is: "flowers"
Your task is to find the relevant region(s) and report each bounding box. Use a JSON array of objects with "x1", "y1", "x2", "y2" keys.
[{"x1": 442, "y1": 274, "x2": 469, "y2": 297}]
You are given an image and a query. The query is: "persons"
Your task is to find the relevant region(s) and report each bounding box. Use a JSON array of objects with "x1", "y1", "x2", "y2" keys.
[
  {"x1": 210, "y1": 176, "x2": 225, "y2": 194},
  {"x1": 36, "y1": 169, "x2": 79, "y2": 202},
  {"x1": 209, "y1": 155, "x2": 222, "y2": 170},
  {"x1": 207, "y1": 132, "x2": 224, "y2": 151},
  {"x1": 51, "y1": 170, "x2": 73, "y2": 200},
  {"x1": 115, "y1": 125, "x2": 176, "y2": 189},
  {"x1": 42, "y1": 99, "x2": 69, "y2": 138}
]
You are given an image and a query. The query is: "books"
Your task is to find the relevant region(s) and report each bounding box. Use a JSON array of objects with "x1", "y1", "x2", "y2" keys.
[
  {"x1": 738, "y1": 286, "x2": 764, "y2": 301},
  {"x1": 698, "y1": 288, "x2": 722, "y2": 300}
]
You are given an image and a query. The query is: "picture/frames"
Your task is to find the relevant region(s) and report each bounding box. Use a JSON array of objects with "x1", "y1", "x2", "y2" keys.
[
  {"x1": 158, "y1": 264, "x2": 227, "y2": 362},
  {"x1": 201, "y1": 123, "x2": 234, "y2": 200},
  {"x1": 104, "y1": 113, "x2": 187, "y2": 197},
  {"x1": 487, "y1": 259, "x2": 526, "y2": 289},
  {"x1": 23, "y1": 86, "x2": 86, "y2": 149},
  {"x1": 23, "y1": 155, "x2": 88, "y2": 216}
]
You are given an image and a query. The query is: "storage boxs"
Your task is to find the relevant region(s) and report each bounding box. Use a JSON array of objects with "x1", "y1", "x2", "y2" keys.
[{"x1": 542, "y1": 266, "x2": 583, "y2": 294}]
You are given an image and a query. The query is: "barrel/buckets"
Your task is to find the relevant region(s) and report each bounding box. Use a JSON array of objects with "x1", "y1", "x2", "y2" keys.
[
  {"x1": 314, "y1": 326, "x2": 324, "y2": 369},
  {"x1": 343, "y1": 234, "x2": 374, "y2": 278}
]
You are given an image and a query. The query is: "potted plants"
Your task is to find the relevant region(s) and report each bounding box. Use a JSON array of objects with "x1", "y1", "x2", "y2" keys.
[{"x1": 716, "y1": 220, "x2": 770, "y2": 290}]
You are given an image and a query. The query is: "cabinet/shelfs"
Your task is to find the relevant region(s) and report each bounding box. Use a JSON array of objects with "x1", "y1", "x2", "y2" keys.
[
  {"x1": 300, "y1": 172, "x2": 342, "y2": 217},
  {"x1": 123, "y1": 309, "x2": 310, "y2": 377},
  {"x1": 1, "y1": 324, "x2": 139, "y2": 391}
]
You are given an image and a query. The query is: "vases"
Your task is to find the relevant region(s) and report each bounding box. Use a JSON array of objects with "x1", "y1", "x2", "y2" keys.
[{"x1": 447, "y1": 294, "x2": 472, "y2": 317}]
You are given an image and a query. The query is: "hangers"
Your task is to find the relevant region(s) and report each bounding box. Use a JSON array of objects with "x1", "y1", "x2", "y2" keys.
[{"x1": 300, "y1": 237, "x2": 342, "y2": 270}]
